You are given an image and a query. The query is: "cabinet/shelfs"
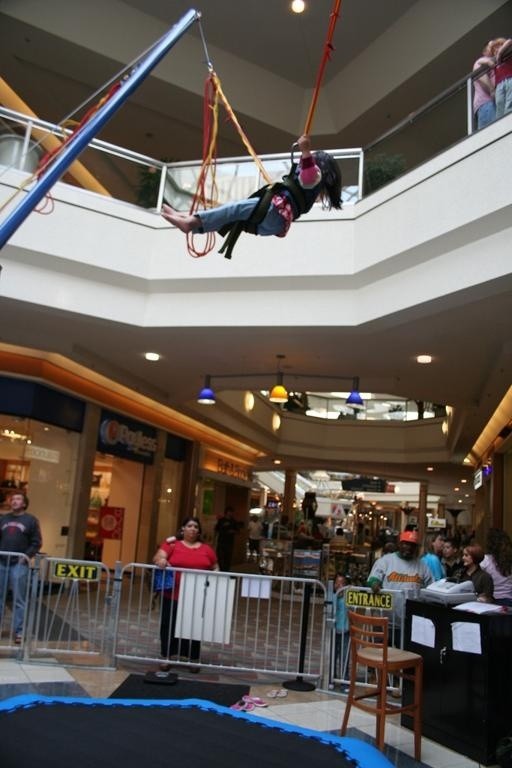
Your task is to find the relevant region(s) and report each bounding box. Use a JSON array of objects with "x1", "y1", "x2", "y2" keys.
[
  {"x1": 321, "y1": 502, "x2": 387, "y2": 587},
  {"x1": 290, "y1": 543, "x2": 322, "y2": 598},
  {"x1": 257, "y1": 538, "x2": 292, "y2": 595},
  {"x1": 400, "y1": 589, "x2": 512, "y2": 768}
]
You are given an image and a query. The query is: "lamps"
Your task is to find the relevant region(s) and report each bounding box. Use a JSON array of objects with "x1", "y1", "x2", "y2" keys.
[
  {"x1": 197, "y1": 374, "x2": 217, "y2": 404},
  {"x1": 344, "y1": 376, "x2": 364, "y2": 410},
  {"x1": 268, "y1": 354, "x2": 289, "y2": 404}
]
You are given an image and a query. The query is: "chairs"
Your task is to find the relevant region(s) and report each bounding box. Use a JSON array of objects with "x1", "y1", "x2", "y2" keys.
[{"x1": 339, "y1": 611, "x2": 422, "y2": 762}]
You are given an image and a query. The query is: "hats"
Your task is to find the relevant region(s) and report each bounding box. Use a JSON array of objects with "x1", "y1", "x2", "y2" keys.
[{"x1": 398, "y1": 530, "x2": 420, "y2": 545}]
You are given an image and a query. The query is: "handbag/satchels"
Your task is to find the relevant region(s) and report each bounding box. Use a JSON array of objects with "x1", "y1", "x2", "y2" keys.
[{"x1": 149, "y1": 566, "x2": 176, "y2": 592}]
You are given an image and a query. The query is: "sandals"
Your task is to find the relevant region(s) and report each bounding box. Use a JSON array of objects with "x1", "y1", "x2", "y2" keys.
[
  {"x1": 266, "y1": 689, "x2": 278, "y2": 698},
  {"x1": 277, "y1": 688, "x2": 288, "y2": 698}
]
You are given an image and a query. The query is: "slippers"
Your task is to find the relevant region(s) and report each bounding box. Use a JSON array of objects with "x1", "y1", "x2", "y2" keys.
[
  {"x1": 241, "y1": 694, "x2": 268, "y2": 708},
  {"x1": 232, "y1": 700, "x2": 255, "y2": 713}
]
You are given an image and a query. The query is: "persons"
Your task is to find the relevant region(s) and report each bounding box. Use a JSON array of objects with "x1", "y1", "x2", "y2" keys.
[
  {"x1": 161, "y1": 134, "x2": 344, "y2": 236},
  {"x1": 367, "y1": 521, "x2": 512, "y2": 698},
  {"x1": 247, "y1": 513, "x2": 263, "y2": 557},
  {"x1": 473, "y1": 40, "x2": 495, "y2": 128},
  {"x1": 1, "y1": 494, "x2": 43, "y2": 644},
  {"x1": 271, "y1": 515, "x2": 350, "y2": 553},
  {"x1": 482, "y1": 37, "x2": 512, "y2": 117},
  {"x1": 153, "y1": 517, "x2": 219, "y2": 673},
  {"x1": 215, "y1": 506, "x2": 241, "y2": 570}
]
